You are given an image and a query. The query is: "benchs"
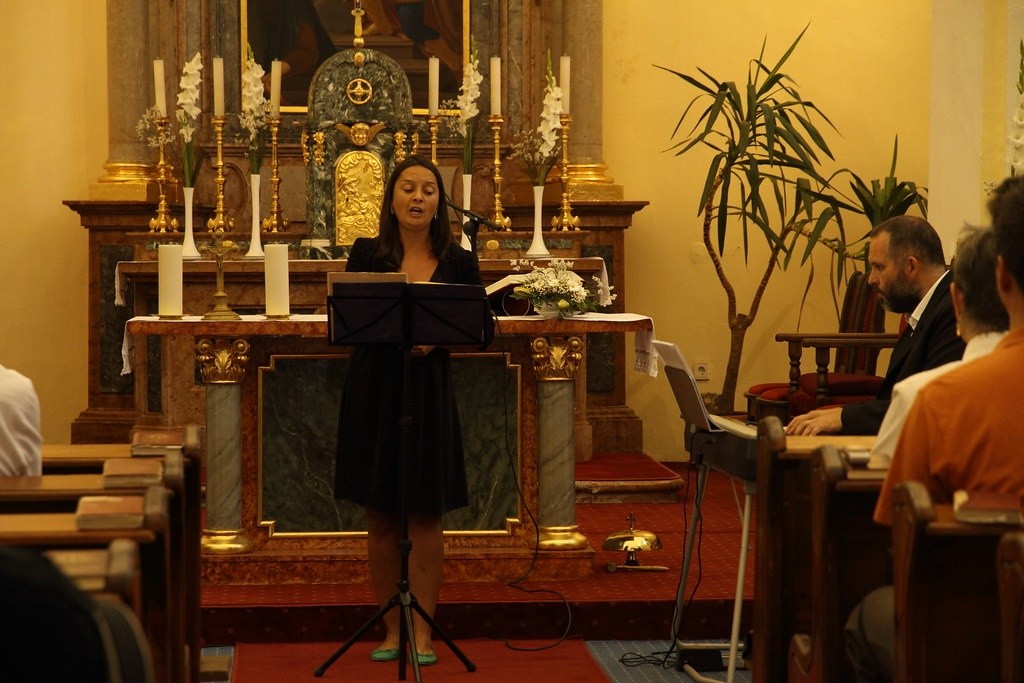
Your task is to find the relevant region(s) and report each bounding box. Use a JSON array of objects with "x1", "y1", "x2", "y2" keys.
[
  {"x1": 755, "y1": 416, "x2": 1023, "y2": 683},
  {"x1": 0, "y1": 444, "x2": 203, "y2": 683}
]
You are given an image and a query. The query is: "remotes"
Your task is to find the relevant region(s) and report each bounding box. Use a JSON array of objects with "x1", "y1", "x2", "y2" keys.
[{"x1": 843, "y1": 445, "x2": 870, "y2": 466}]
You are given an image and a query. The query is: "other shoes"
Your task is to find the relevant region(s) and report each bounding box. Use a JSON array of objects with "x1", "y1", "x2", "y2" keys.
[
  {"x1": 371, "y1": 649, "x2": 399, "y2": 661},
  {"x1": 410, "y1": 644, "x2": 436, "y2": 665}
]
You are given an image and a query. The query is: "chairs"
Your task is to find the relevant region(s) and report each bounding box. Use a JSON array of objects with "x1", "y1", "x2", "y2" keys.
[{"x1": 745, "y1": 271, "x2": 905, "y2": 422}]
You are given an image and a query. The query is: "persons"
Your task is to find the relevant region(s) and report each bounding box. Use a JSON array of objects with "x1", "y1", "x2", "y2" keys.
[
  {"x1": 333, "y1": 155, "x2": 494, "y2": 665},
  {"x1": 0, "y1": 543, "x2": 156, "y2": 683},
  {"x1": 786, "y1": 215, "x2": 967, "y2": 439},
  {"x1": 827, "y1": 226, "x2": 1010, "y2": 471},
  {"x1": 844, "y1": 174, "x2": 1024, "y2": 683},
  {"x1": 0, "y1": 364, "x2": 43, "y2": 477}
]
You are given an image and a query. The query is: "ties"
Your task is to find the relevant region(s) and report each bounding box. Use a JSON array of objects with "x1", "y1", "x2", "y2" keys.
[{"x1": 891, "y1": 323, "x2": 914, "y2": 366}]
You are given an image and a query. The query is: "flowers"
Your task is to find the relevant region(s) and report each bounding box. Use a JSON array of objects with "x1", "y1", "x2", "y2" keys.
[
  {"x1": 506, "y1": 50, "x2": 562, "y2": 186},
  {"x1": 507, "y1": 260, "x2": 617, "y2": 314},
  {"x1": 136, "y1": 53, "x2": 216, "y2": 188},
  {"x1": 235, "y1": 38, "x2": 271, "y2": 175},
  {"x1": 439, "y1": 35, "x2": 485, "y2": 174}
]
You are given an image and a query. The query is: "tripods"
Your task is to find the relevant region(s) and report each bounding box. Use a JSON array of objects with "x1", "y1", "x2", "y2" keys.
[{"x1": 314, "y1": 271, "x2": 493, "y2": 683}]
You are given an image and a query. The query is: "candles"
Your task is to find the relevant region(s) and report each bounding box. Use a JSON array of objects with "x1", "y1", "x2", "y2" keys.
[
  {"x1": 560, "y1": 53, "x2": 570, "y2": 114},
  {"x1": 265, "y1": 244, "x2": 290, "y2": 315},
  {"x1": 213, "y1": 55, "x2": 225, "y2": 116},
  {"x1": 271, "y1": 57, "x2": 281, "y2": 118},
  {"x1": 154, "y1": 57, "x2": 167, "y2": 117},
  {"x1": 428, "y1": 55, "x2": 440, "y2": 114},
  {"x1": 158, "y1": 244, "x2": 183, "y2": 315},
  {"x1": 490, "y1": 54, "x2": 501, "y2": 115}
]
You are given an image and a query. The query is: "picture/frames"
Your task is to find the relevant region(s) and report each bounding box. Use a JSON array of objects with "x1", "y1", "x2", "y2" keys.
[{"x1": 240, "y1": 0, "x2": 470, "y2": 114}]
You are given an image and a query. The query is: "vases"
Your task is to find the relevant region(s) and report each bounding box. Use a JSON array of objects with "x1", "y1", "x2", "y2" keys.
[
  {"x1": 525, "y1": 187, "x2": 551, "y2": 254},
  {"x1": 245, "y1": 175, "x2": 264, "y2": 256},
  {"x1": 460, "y1": 174, "x2": 471, "y2": 251},
  {"x1": 534, "y1": 303, "x2": 563, "y2": 318},
  {"x1": 182, "y1": 188, "x2": 201, "y2": 257}
]
